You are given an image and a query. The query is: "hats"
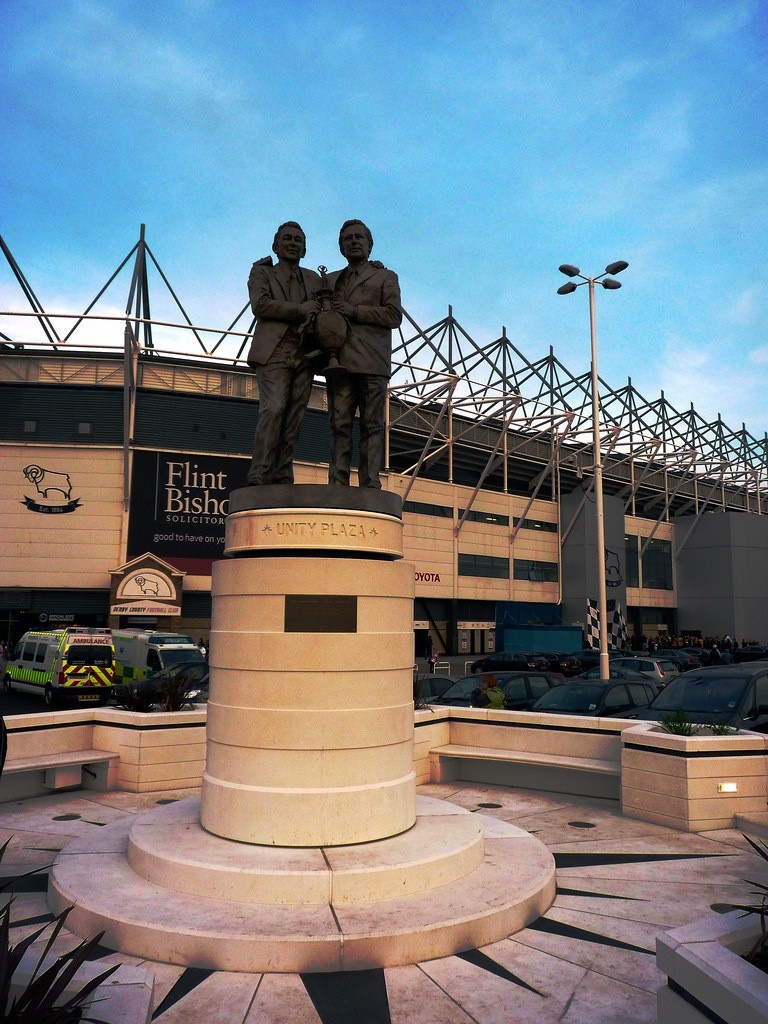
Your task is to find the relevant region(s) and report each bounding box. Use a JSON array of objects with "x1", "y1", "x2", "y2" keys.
[{"x1": 713, "y1": 645, "x2": 717, "y2": 648}]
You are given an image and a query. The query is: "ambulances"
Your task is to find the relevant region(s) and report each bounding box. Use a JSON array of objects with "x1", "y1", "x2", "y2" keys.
[
  {"x1": 110, "y1": 628, "x2": 207, "y2": 700},
  {"x1": 1, "y1": 626, "x2": 116, "y2": 707}
]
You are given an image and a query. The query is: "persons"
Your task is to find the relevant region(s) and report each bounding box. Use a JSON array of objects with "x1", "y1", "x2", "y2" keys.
[
  {"x1": 470, "y1": 674, "x2": 508, "y2": 709},
  {"x1": 424, "y1": 635, "x2": 439, "y2": 674},
  {"x1": 0, "y1": 640, "x2": 15, "y2": 660},
  {"x1": 193, "y1": 636, "x2": 209, "y2": 658},
  {"x1": 247, "y1": 219, "x2": 402, "y2": 489},
  {"x1": 625, "y1": 630, "x2": 758, "y2": 665}
]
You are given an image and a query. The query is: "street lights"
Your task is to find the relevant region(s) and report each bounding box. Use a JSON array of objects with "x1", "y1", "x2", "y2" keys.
[{"x1": 555, "y1": 259, "x2": 632, "y2": 679}]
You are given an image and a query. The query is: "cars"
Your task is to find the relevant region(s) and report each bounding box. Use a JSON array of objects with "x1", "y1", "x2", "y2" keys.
[{"x1": 413, "y1": 642, "x2": 768, "y2": 734}]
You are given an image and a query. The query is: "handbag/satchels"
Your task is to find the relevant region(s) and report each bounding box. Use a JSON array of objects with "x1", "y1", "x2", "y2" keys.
[{"x1": 428, "y1": 656, "x2": 432, "y2": 664}]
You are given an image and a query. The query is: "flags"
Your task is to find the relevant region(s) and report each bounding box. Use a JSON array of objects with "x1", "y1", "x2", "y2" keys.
[{"x1": 587, "y1": 599, "x2": 632, "y2": 649}]
